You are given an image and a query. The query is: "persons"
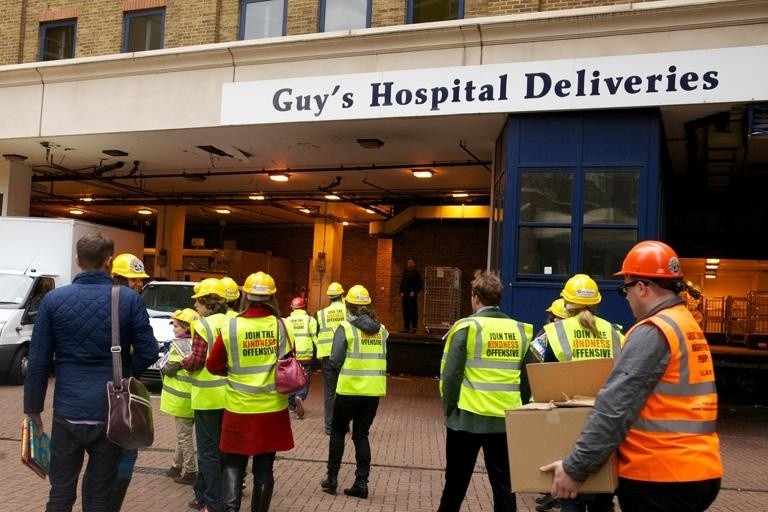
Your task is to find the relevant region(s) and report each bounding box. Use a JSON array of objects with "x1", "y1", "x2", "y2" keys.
[
  {"x1": 159, "y1": 271, "x2": 296, "y2": 511},
  {"x1": 321, "y1": 285, "x2": 390, "y2": 497},
  {"x1": 108, "y1": 251, "x2": 151, "y2": 511},
  {"x1": 435, "y1": 269, "x2": 534, "y2": 511},
  {"x1": 679, "y1": 281, "x2": 704, "y2": 330},
  {"x1": 398, "y1": 259, "x2": 423, "y2": 334},
  {"x1": 314, "y1": 282, "x2": 350, "y2": 435},
  {"x1": 531, "y1": 273, "x2": 626, "y2": 363},
  {"x1": 539, "y1": 240, "x2": 725, "y2": 511},
  {"x1": 23, "y1": 232, "x2": 157, "y2": 511},
  {"x1": 287, "y1": 296, "x2": 318, "y2": 419}
]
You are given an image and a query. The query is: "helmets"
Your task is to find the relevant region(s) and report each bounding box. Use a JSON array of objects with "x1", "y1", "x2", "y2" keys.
[
  {"x1": 243, "y1": 271, "x2": 277, "y2": 295},
  {"x1": 560, "y1": 274, "x2": 602, "y2": 305},
  {"x1": 326, "y1": 281, "x2": 345, "y2": 296},
  {"x1": 170, "y1": 308, "x2": 199, "y2": 323},
  {"x1": 345, "y1": 284, "x2": 371, "y2": 305},
  {"x1": 611, "y1": 240, "x2": 684, "y2": 280},
  {"x1": 111, "y1": 252, "x2": 151, "y2": 280},
  {"x1": 545, "y1": 298, "x2": 568, "y2": 319},
  {"x1": 191, "y1": 276, "x2": 241, "y2": 300},
  {"x1": 290, "y1": 297, "x2": 306, "y2": 308}
]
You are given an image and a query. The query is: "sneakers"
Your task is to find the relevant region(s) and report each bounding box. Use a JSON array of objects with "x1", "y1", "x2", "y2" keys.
[
  {"x1": 398, "y1": 328, "x2": 417, "y2": 334},
  {"x1": 344, "y1": 482, "x2": 369, "y2": 498},
  {"x1": 320, "y1": 475, "x2": 338, "y2": 492},
  {"x1": 289, "y1": 406, "x2": 296, "y2": 413},
  {"x1": 175, "y1": 472, "x2": 198, "y2": 485},
  {"x1": 534, "y1": 495, "x2": 560, "y2": 512},
  {"x1": 164, "y1": 467, "x2": 182, "y2": 477},
  {"x1": 295, "y1": 396, "x2": 305, "y2": 420}
]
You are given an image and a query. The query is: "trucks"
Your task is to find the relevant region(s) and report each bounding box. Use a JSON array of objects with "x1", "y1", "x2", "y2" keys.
[{"x1": 0, "y1": 215, "x2": 146, "y2": 384}]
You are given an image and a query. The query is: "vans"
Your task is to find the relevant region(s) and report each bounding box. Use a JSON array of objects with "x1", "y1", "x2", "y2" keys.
[{"x1": 136, "y1": 278, "x2": 281, "y2": 383}]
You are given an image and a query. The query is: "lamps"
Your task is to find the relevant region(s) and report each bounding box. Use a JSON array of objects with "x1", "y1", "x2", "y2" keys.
[{"x1": 66, "y1": 168, "x2": 434, "y2": 217}]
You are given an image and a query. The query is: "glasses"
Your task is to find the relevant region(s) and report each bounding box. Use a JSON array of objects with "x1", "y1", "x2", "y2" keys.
[{"x1": 616, "y1": 281, "x2": 649, "y2": 296}]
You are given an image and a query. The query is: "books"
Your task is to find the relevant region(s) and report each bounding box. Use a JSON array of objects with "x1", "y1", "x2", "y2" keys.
[{"x1": 21, "y1": 416, "x2": 50, "y2": 480}]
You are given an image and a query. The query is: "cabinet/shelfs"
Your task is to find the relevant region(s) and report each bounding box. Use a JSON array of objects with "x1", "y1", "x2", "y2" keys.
[{"x1": 698, "y1": 287, "x2": 768, "y2": 349}]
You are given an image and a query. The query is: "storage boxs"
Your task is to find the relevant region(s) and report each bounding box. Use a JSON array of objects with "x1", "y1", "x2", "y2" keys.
[{"x1": 503, "y1": 356, "x2": 622, "y2": 495}]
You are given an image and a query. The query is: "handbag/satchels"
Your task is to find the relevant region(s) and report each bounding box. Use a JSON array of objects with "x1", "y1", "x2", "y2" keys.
[
  {"x1": 274, "y1": 316, "x2": 312, "y2": 395},
  {"x1": 106, "y1": 374, "x2": 154, "y2": 448}
]
